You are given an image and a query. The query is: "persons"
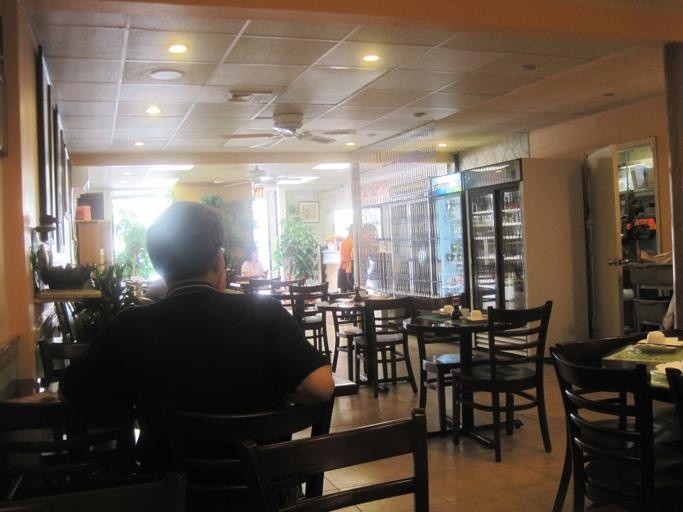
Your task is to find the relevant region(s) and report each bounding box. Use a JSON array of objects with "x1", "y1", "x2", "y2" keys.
[
  {"x1": 347, "y1": 223, "x2": 375, "y2": 288},
  {"x1": 337, "y1": 224, "x2": 353, "y2": 321},
  {"x1": 61, "y1": 200, "x2": 335, "y2": 512}
]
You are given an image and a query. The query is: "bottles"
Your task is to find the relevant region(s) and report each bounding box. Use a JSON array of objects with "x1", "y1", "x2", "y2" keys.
[
  {"x1": 353, "y1": 287, "x2": 363, "y2": 303},
  {"x1": 450, "y1": 297, "x2": 463, "y2": 320}
]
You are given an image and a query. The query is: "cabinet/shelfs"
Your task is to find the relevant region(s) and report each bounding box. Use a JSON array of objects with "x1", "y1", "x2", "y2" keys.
[
  {"x1": 77, "y1": 219, "x2": 100, "y2": 270},
  {"x1": 100, "y1": 220, "x2": 112, "y2": 266}
]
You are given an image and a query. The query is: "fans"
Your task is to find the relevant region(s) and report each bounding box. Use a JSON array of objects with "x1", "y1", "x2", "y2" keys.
[
  {"x1": 222, "y1": 168, "x2": 302, "y2": 189},
  {"x1": 221, "y1": 104, "x2": 357, "y2": 151}
]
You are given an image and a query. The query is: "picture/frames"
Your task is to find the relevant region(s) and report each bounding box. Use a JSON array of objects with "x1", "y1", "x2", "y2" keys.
[{"x1": 298, "y1": 200, "x2": 320, "y2": 223}]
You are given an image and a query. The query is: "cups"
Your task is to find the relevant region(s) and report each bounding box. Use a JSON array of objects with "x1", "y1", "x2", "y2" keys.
[
  {"x1": 444, "y1": 304, "x2": 454, "y2": 313},
  {"x1": 646, "y1": 331, "x2": 666, "y2": 345},
  {"x1": 471, "y1": 310, "x2": 481, "y2": 319}
]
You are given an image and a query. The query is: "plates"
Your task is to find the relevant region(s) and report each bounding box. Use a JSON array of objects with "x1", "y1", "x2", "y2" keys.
[
  {"x1": 468, "y1": 316, "x2": 486, "y2": 320},
  {"x1": 638, "y1": 337, "x2": 681, "y2": 352}
]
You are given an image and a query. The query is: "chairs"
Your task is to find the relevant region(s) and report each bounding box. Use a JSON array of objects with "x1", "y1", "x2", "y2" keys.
[
  {"x1": 453, "y1": 300, "x2": 554, "y2": 463},
  {"x1": 355, "y1": 296, "x2": 418, "y2": 398},
  {"x1": 38, "y1": 341, "x2": 96, "y2": 455},
  {"x1": 289, "y1": 283, "x2": 331, "y2": 367},
  {"x1": 0, "y1": 475, "x2": 191, "y2": 512},
  {"x1": 240, "y1": 406, "x2": 429, "y2": 512},
  {"x1": 271, "y1": 279, "x2": 304, "y2": 308},
  {"x1": 634, "y1": 329, "x2": 683, "y2": 343},
  {"x1": 249, "y1": 277, "x2": 282, "y2": 296},
  {"x1": 549, "y1": 346, "x2": 683, "y2": 511},
  {"x1": 161, "y1": 395, "x2": 334, "y2": 512},
  {"x1": 550, "y1": 335, "x2": 635, "y2": 512},
  {"x1": 0, "y1": 388, "x2": 136, "y2": 500},
  {"x1": 328, "y1": 288, "x2": 366, "y2": 374},
  {"x1": 665, "y1": 367, "x2": 683, "y2": 439},
  {"x1": 409, "y1": 294, "x2": 490, "y2": 439}
]
